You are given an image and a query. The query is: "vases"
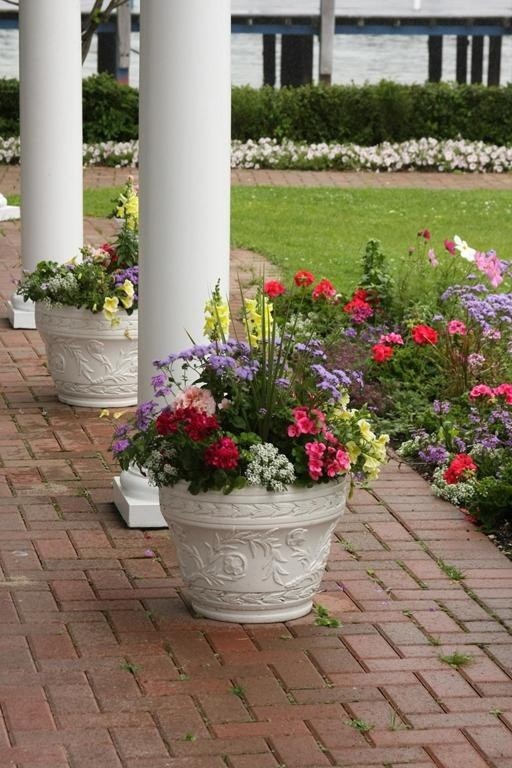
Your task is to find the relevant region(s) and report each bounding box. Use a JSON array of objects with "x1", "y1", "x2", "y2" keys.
[
  {"x1": 157, "y1": 469, "x2": 351, "y2": 624},
  {"x1": 34, "y1": 300, "x2": 139, "y2": 408}
]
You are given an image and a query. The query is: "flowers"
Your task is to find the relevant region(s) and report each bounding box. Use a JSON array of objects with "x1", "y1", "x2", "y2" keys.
[
  {"x1": 114, "y1": 270, "x2": 392, "y2": 492},
  {"x1": 17, "y1": 176, "x2": 138, "y2": 327}
]
